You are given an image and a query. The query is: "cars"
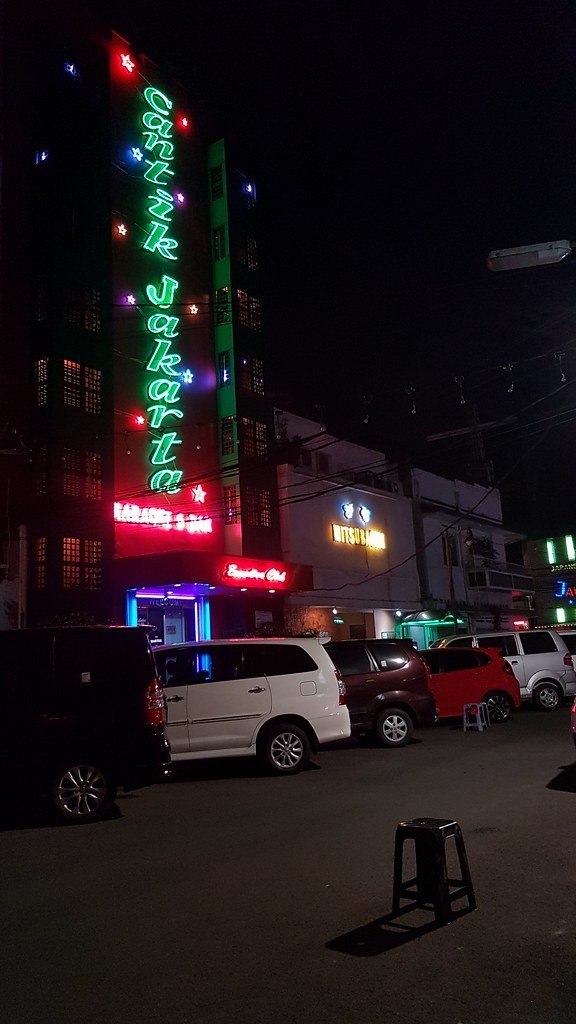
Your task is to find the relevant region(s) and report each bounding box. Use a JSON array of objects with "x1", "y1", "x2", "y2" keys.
[
  {"x1": 557, "y1": 631, "x2": 576, "y2": 672},
  {"x1": 419, "y1": 648, "x2": 522, "y2": 724},
  {"x1": 150, "y1": 637, "x2": 351, "y2": 776},
  {"x1": 322, "y1": 640, "x2": 437, "y2": 746},
  {"x1": 431, "y1": 630, "x2": 576, "y2": 710}
]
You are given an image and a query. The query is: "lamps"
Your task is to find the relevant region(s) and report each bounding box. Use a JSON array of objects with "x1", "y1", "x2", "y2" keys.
[{"x1": 463, "y1": 526, "x2": 474, "y2": 547}]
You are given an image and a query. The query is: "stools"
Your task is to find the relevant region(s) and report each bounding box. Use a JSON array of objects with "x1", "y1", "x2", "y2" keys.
[
  {"x1": 391, "y1": 817, "x2": 477, "y2": 927},
  {"x1": 463, "y1": 702, "x2": 491, "y2": 733}
]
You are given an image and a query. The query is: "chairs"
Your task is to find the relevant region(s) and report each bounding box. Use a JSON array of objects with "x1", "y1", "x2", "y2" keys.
[
  {"x1": 166, "y1": 660, "x2": 176, "y2": 676},
  {"x1": 168, "y1": 653, "x2": 195, "y2": 686}
]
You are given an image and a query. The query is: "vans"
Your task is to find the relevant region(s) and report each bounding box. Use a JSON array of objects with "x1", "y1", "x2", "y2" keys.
[{"x1": 0, "y1": 626, "x2": 171, "y2": 821}]
